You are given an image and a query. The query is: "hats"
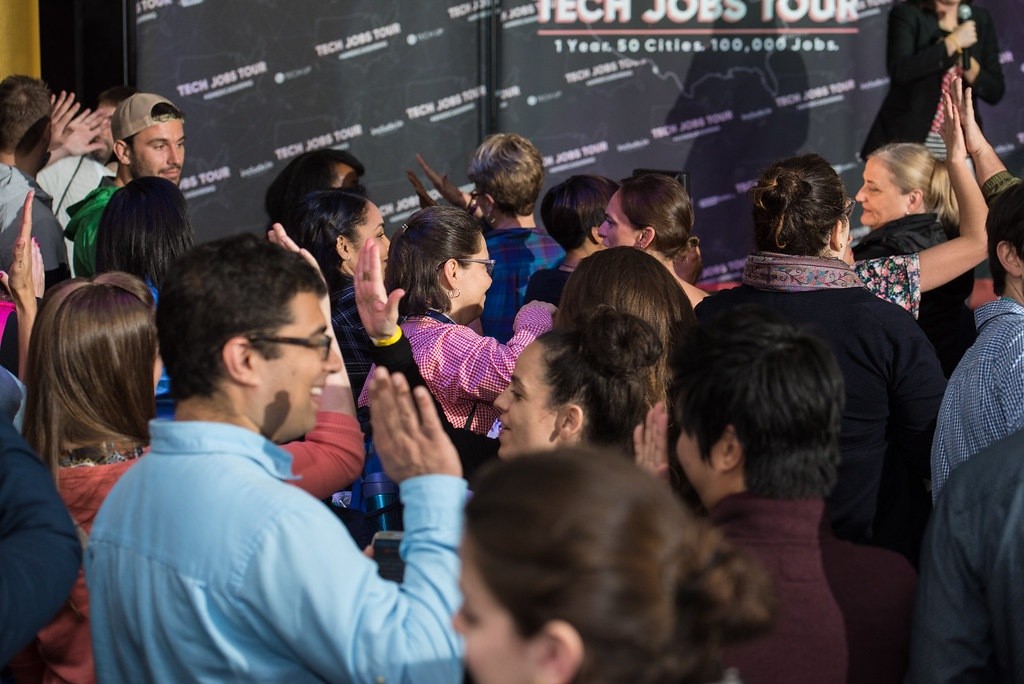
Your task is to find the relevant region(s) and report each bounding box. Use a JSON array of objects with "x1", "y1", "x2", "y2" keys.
[{"x1": 104, "y1": 92, "x2": 184, "y2": 167}]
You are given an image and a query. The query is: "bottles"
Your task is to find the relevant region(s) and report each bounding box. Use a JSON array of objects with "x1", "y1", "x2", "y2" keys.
[{"x1": 360, "y1": 471, "x2": 403, "y2": 542}]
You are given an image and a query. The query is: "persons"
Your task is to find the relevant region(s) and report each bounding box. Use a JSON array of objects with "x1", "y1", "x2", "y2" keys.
[
  {"x1": 859, "y1": 0, "x2": 1004, "y2": 178},
  {"x1": 0, "y1": 73, "x2": 1023, "y2": 684}
]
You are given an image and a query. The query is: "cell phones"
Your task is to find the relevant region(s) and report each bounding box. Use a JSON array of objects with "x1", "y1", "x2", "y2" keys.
[{"x1": 370, "y1": 531, "x2": 406, "y2": 585}]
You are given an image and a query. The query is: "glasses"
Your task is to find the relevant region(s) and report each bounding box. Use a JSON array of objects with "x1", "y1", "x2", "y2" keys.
[
  {"x1": 212, "y1": 335, "x2": 333, "y2": 362},
  {"x1": 437, "y1": 259, "x2": 496, "y2": 274},
  {"x1": 469, "y1": 189, "x2": 493, "y2": 201}
]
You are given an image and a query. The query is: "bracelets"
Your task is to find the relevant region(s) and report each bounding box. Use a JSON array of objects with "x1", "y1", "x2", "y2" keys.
[{"x1": 948, "y1": 34, "x2": 963, "y2": 54}]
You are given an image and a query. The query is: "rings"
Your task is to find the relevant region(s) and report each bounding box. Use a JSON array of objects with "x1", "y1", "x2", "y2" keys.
[{"x1": 363, "y1": 271, "x2": 370, "y2": 281}]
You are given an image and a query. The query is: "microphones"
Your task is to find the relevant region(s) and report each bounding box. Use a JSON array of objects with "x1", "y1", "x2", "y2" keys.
[{"x1": 958, "y1": 4, "x2": 972, "y2": 71}]
